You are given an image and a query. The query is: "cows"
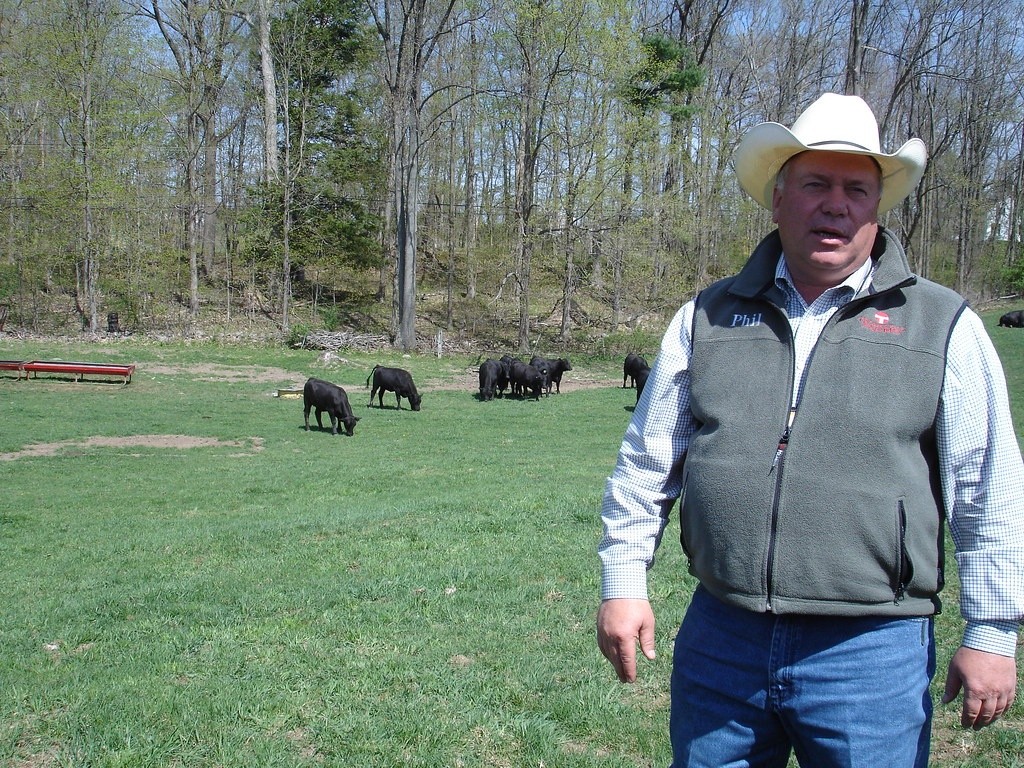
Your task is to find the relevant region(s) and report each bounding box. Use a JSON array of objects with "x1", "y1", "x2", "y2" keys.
[
  {"x1": 479, "y1": 355, "x2": 573, "y2": 402},
  {"x1": 303, "y1": 377, "x2": 362, "y2": 437},
  {"x1": 623, "y1": 352, "x2": 652, "y2": 401},
  {"x1": 996, "y1": 310, "x2": 1024, "y2": 328},
  {"x1": 366, "y1": 363, "x2": 424, "y2": 411}
]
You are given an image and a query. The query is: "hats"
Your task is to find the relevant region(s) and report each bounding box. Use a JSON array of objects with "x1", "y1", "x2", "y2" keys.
[{"x1": 732, "y1": 92, "x2": 927, "y2": 216}]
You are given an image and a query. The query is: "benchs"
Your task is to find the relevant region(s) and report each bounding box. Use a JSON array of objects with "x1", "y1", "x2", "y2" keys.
[{"x1": 0, "y1": 360, "x2": 136, "y2": 387}]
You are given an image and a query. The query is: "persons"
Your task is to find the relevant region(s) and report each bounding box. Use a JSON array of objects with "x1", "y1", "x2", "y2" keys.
[{"x1": 597, "y1": 92, "x2": 1024, "y2": 767}]
